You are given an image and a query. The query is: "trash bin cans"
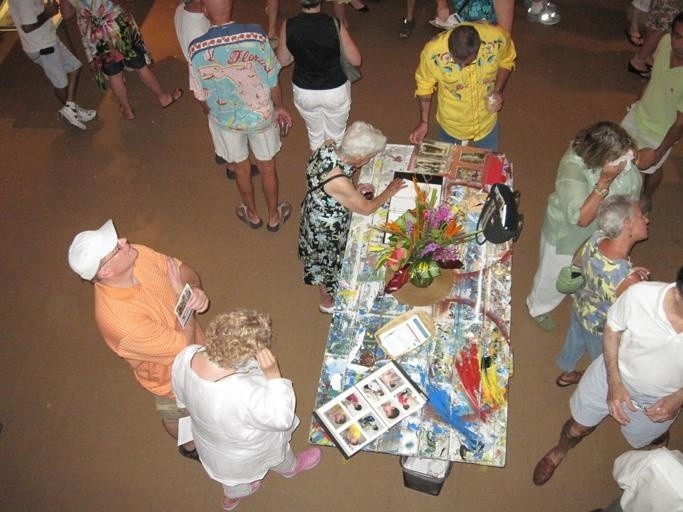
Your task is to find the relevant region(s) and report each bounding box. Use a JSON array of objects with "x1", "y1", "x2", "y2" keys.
[{"x1": 399, "y1": 454, "x2": 453, "y2": 498}]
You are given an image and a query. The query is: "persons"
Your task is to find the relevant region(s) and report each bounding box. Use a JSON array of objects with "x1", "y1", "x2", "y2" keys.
[
  {"x1": 531, "y1": 267, "x2": 683, "y2": 487},
  {"x1": 589, "y1": 448, "x2": 683, "y2": 512},
  {"x1": 324, "y1": 368, "x2": 423, "y2": 454},
  {"x1": 527, "y1": 0, "x2": 560, "y2": 25},
  {"x1": 168, "y1": 309, "x2": 322, "y2": 512},
  {"x1": 278, "y1": 0, "x2": 363, "y2": 155},
  {"x1": 352, "y1": 0, "x2": 370, "y2": 13},
  {"x1": 174, "y1": 0, "x2": 293, "y2": 229},
  {"x1": 521, "y1": 120, "x2": 644, "y2": 331},
  {"x1": 400, "y1": 0, "x2": 517, "y2": 153},
  {"x1": 60, "y1": 0, "x2": 184, "y2": 120},
  {"x1": 9, "y1": 0, "x2": 97, "y2": 131},
  {"x1": 64, "y1": 218, "x2": 209, "y2": 440},
  {"x1": 621, "y1": 0, "x2": 683, "y2": 191},
  {"x1": 555, "y1": 193, "x2": 652, "y2": 390},
  {"x1": 294, "y1": 120, "x2": 410, "y2": 315}
]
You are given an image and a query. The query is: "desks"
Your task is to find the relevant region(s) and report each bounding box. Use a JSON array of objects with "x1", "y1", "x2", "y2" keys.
[{"x1": 305, "y1": 139, "x2": 514, "y2": 469}]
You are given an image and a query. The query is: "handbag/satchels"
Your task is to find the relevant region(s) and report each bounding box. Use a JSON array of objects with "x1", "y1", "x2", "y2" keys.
[
  {"x1": 475, "y1": 183, "x2": 525, "y2": 249},
  {"x1": 340, "y1": 55, "x2": 363, "y2": 86},
  {"x1": 556, "y1": 265, "x2": 586, "y2": 295}
]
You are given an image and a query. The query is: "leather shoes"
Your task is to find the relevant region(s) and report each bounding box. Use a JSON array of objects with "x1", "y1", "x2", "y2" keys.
[
  {"x1": 348, "y1": 1, "x2": 370, "y2": 13},
  {"x1": 226, "y1": 163, "x2": 259, "y2": 179},
  {"x1": 533, "y1": 444, "x2": 566, "y2": 486},
  {"x1": 317, "y1": 301, "x2": 335, "y2": 313},
  {"x1": 214, "y1": 154, "x2": 226, "y2": 164}
]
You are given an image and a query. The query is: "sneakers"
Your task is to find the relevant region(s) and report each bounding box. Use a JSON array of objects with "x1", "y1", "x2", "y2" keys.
[
  {"x1": 58, "y1": 106, "x2": 85, "y2": 132},
  {"x1": 76, "y1": 106, "x2": 97, "y2": 123},
  {"x1": 525, "y1": 7, "x2": 560, "y2": 26},
  {"x1": 275, "y1": 445, "x2": 323, "y2": 479},
  {"x1": 533, "y1": 313, "x2": 554, "y2": 330},
  {"x1": 220, "y1": 480, "x2": 264, "y2": 511}
]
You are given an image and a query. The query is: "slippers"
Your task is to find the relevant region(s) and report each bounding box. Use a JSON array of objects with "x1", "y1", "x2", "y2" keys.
[
  {"x1": 236, "y1": 203, "x2": 263, "y2": 229},
  {"x1": 629, "y1": 62, "x2": 651, "y2": 80},
  {"x1": 265, "y1": 200, "x2": 292, "y2": 232},
  {"x1": 624, "y1": 27, "x2": 645, "y2": 48},
  {"x1": 118, "y1": 103, "x2": 136, "y2": 121},
  {"x1": 162, "y1": 87, "x2": 184, "y2": 108}
]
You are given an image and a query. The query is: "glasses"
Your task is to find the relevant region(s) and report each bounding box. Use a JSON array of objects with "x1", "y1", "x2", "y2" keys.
[{"x1": 102, "y1": 243, "x2": 123, "y2": 264}]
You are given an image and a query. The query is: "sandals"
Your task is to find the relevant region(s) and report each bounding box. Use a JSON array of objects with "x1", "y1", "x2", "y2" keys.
[
  {"x1": 398, "y1": 17, "x2": 415, "y2": 38},
  {"x1": 556, "y1": 370, "x2": 585, "y2": 388},
  {"x1": 178, "y1": 441, "x2": 201, "y2": 461}
]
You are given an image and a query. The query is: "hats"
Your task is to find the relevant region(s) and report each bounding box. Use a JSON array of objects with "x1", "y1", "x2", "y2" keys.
[{"x1": 66, "y1": 218, "x2": 118, "y2": 282}]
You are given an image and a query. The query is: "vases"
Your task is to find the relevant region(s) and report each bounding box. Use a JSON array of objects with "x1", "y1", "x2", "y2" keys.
[{"x1": 381, "y1": 263, "x2": 455, "y2": 305}]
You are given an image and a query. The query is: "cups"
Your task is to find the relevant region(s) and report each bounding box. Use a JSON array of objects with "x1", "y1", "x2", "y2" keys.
[
  {"x1": 277, "y1": 117, "x2": 288, "y2": 136},
  {"x1": 484, "y1": 94, "x2": 497, "y2": 111}
]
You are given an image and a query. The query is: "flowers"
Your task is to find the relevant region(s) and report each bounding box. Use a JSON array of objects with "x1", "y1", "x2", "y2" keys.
[{"x1": 362, "y1": 169, "x2": 487, "y2": 291}]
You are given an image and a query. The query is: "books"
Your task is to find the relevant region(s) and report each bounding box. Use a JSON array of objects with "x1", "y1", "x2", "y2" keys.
[{"x1": 312, "y1": 359, "x2": 431, "y2": 460}]
